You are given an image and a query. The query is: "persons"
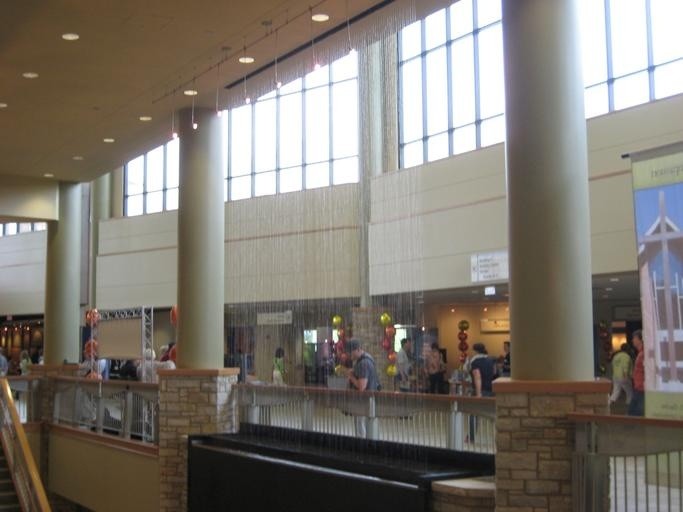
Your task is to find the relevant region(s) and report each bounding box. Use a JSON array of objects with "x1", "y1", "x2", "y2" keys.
[
  {"x1": 272, "y1": 337, "x2": 510, "y2": 441},
  {"x1": 608, "y1": 343, "x2": 634, "y2": 406},
  {"x1": 14, "y1": 351, "x2": 34, "y2": 376},
  {"x1": 136, "y1": 349, "x2": 159, "y2": 384},
  {"x1": 628, "y1": 330, "x2": 644, "y2": 416},
  {"x1": 0, "y1": 354, "x2": 8, "y2": 377}
]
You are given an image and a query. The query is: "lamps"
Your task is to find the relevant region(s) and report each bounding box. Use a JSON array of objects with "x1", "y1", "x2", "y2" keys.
[
  {"x1": 310, "y1": 9, "x2": 320, "y2": 71},
  {"x1": 346, "y1": 0, "x2": 356, "y2": 56},
  {"x1": 244, "y1": 49, "x2": 251, "y2": 104},
  {"x1": 171, "y1": 94, "x2": 178, "y2": 140},
  {"x1": 192, "y1": 79, "x2": 197, "y2": 130},
  {"x1": 274, "y1": 31, "x2": 281, "y2": 88},
  {"x1": 216, "y1": 64, "x2": 222, "y2": 117}
]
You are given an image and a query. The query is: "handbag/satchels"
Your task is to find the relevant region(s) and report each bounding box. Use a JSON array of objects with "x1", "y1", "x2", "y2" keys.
[{"x1": 282, "y1": 371, "x2": 289, "y2": 382}]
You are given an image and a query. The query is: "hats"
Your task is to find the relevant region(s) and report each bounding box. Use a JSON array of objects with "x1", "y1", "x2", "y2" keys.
[{"x1": 343, "y1": 340, "x2": 360, "y2": 353}]
